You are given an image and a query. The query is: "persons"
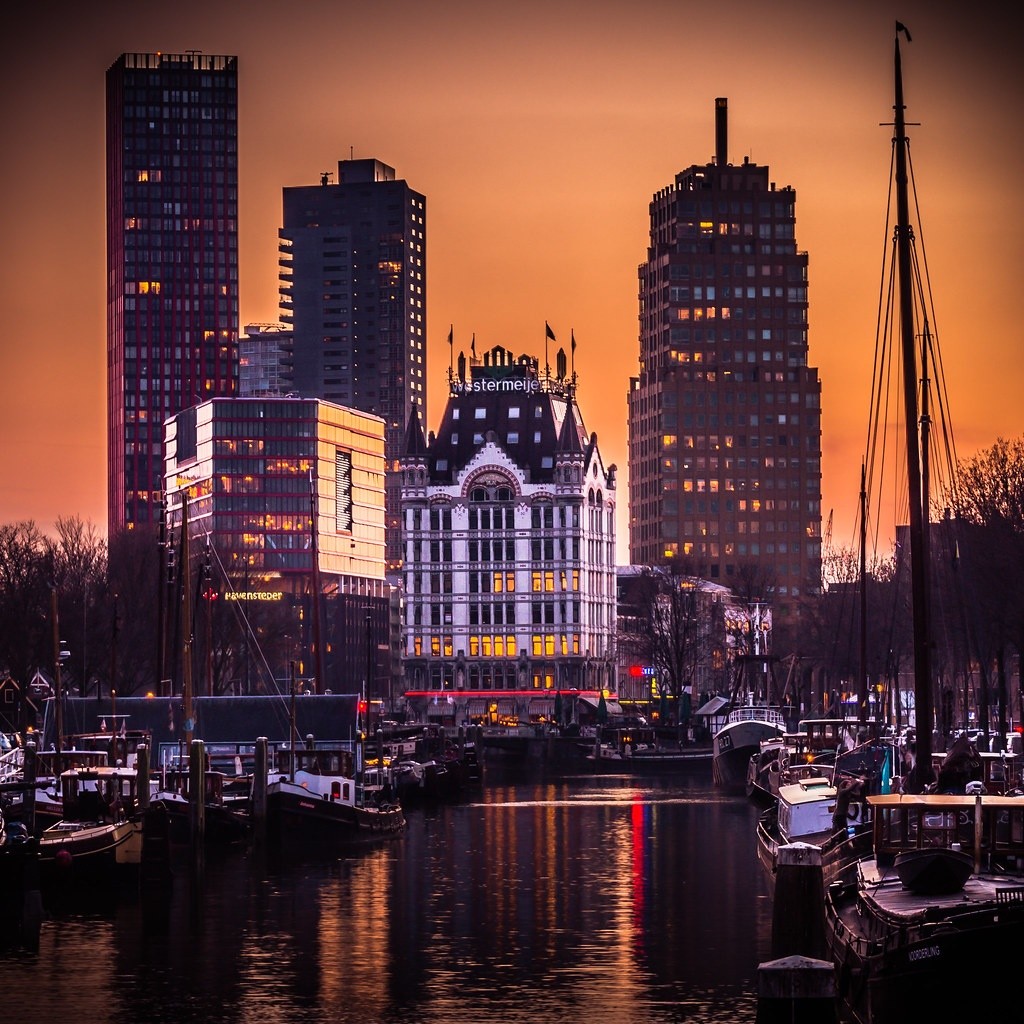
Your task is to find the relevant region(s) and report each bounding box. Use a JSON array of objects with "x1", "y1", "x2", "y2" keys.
[{"x1": 832, "y1": 779, "x2": 861, "y2": 844}]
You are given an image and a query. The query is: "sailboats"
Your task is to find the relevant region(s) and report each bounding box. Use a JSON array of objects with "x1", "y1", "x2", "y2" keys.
[
  {"x1": 519, "y1": 20, "x2": 1024, "y2": 1023},
  {"x1": 0, "y1": 485, "x2": 485, "y2": 898}
]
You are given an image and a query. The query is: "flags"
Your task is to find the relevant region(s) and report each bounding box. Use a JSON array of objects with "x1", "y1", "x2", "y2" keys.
[
  {"x1": 573, "y1": 335, "x2": 577, "y2": 350},
  {"x1": 547, "y1": 325, "x2": 557, "y2": 341},
  {"x1": 447, "y1": 331, "x2": 451, "y2": 344}
]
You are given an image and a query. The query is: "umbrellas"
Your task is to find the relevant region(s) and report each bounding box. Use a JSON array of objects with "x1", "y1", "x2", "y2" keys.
[
  {"x1": 698, "y1": 692, "x2": 707, "y2": 724},
  {"x1": 679, "y1": 692, "x2": 690, "y2": 721},
  {"x1": 658, "y1": 690, "x2": 669, "y2": 718},
  {"x1": 554, "y1": 690, "x2": 562, "y2": 723},
  {"x1": 596, "y1": 692, "x2": 608, "y2": 723}
]
